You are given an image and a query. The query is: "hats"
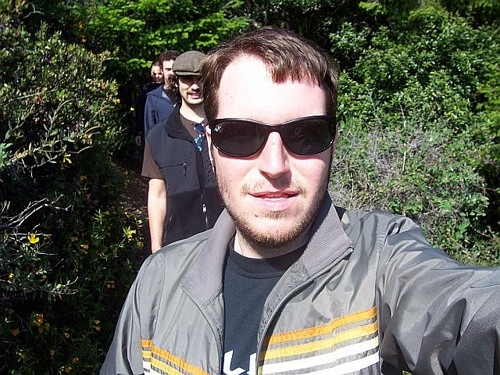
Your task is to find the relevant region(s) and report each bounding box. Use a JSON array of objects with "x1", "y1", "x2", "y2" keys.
[{"x1": 172, "y1": 50, "x2": 206, "y2": 75}]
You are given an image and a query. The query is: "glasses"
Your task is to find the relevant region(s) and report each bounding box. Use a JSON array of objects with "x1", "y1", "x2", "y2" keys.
[{"x1": 209, "y1": 115, "x2": 337, "y2": 157}]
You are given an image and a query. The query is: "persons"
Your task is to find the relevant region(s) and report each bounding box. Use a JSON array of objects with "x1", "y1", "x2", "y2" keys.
[
  {"x1": 141, "y1": 50, "x2": 224, "y2": 256},
  {"x1": 99, "y1": 26, "x2": 500, "y2": 375}
]
